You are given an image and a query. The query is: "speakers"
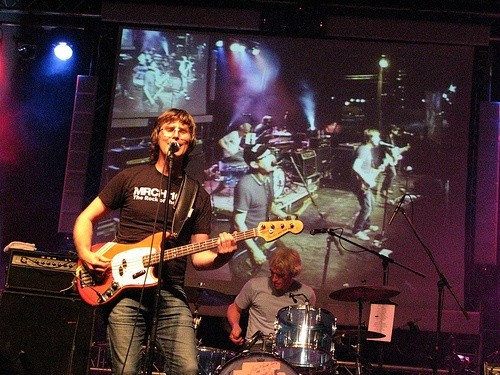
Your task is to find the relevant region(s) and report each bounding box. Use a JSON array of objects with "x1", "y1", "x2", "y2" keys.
[
  {"x1": 292, "y1": 149, "x2": 317, "y2": 177},
  {"x1": 477, "y1": 299, "x2": 500, "y2": 375},
  {"x1": 0, "y1": 289, "x2": 94, "y2": 375}
]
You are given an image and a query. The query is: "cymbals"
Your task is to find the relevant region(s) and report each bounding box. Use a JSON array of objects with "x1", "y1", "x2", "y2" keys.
[
  {"x1": 329, "y1": 285, "x2": 402, "y2": 302},
  {"x1": 335, "y1": 329, "x2": 387, "y2": 338},
  {"x1": 183, "y1": 286, "x2": 233, "y2": 306}
]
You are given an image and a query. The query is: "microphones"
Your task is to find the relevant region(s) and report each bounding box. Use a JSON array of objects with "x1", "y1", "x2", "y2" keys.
[
  {"x1": 17, "y1": 350, "x2": 26, "y2": 357},
  {"x1": 388, "y1": 196, "x2": 405, "y2": 226},
  {"x1": 271, "y1": 151, "x2": 292, "y2": 166},
  {"x1": 170, "y1": 142, "x2": 180, "y2": 153},
  {"x1": 379, "y1": 141, "x2": 394, "y2": 148},
  {"x1": 310, "y1": 228, "x2": 334, "y2": 236}
]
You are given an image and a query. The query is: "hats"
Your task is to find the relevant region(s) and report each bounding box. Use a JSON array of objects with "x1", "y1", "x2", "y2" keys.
[{"x1": 243, "y1": 141, "x2": 275, "y2": 164}]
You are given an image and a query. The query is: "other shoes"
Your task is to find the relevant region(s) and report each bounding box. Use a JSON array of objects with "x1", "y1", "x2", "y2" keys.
[
  {"x1": 367, "y1": 225, "x2": 379, "y2": 231},
  {"x1": 351, "y1": 231, "x2": 370, "y2": 241}
]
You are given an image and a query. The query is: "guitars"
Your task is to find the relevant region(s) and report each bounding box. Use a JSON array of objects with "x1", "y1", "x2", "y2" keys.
[
  {"x1": 385, "y1": 143, "x2": 410, "y2": 165},
  {"x1": 230, "y1": 194, "x2": 318, "y2": 281},
  {"x1": 76, "y1": 215, "x2": 305, "y2": 307},
  {"x1": 143, "y1": 81, "x2": 167, "y2": 113}
]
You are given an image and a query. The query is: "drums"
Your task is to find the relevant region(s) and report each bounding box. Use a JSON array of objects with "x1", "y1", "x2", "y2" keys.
[
  {"x1": 198, "y1": 346, "x2": 236, "y2": 375},
  {"x1": 211, "y1": 350, "x2": 301, "y2": 375},
  {"x1": 272, "y1": 305, "x2": 335, "y2": 367}
]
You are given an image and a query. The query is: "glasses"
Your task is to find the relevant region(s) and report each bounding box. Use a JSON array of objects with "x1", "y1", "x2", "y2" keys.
[{"x1": 159, "y1": 128, "x2": 191, "y2": 139}]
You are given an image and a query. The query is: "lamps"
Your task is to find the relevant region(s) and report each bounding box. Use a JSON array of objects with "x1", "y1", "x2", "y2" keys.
[{"x1": 16, "y1": 39, "x2": 37, "y2": 60}]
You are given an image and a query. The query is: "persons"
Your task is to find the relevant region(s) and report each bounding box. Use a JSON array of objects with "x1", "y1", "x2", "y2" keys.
[
  {"x1": 73, "y1": 108, "x2": 239, "y2": 375},
  {"x1": 255, "y1": 115, "x2": 274, "y2": 144},
  {"x1": 227, "y1": 247, "x2": 315, "y2": 348},
  {"x1": 132, "y1": 47, "x2": 197, "y2": 112},
  {"x1": 351, "y1": 130, "x2": 385, "y2": 241},
  {"x1": 229, "y1": 144, "x2": 294, "y2": 283},
  {"x1": 217, "y1": 123, "x2": 250, "y2": 162},
  {"x1": 380, "y1": 124, "x2": 408, "y2": 197}
]
